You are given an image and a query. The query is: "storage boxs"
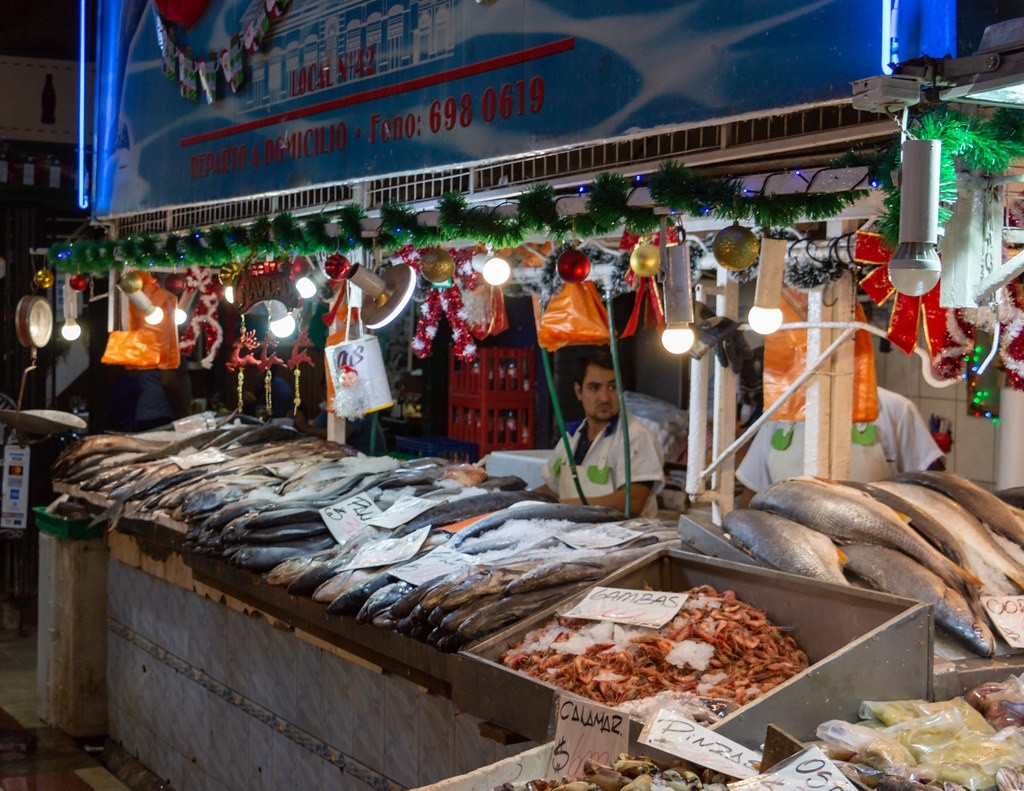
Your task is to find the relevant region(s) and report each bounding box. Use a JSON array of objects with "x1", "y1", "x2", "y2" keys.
[
  {"x1": 394, "y1": 344, "x2": 565, "y2": 492},
  {"x1": 30, "y1": 503, "x2": 99, "y2": 541},
  {"x1": 458, "y1": 548, "x2": 933, "y2": 766}
]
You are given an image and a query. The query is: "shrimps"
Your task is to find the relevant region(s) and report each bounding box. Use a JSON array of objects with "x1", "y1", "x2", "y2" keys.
[{"x1": 507, "y1": 584, "x2": 811, "y2": 731}]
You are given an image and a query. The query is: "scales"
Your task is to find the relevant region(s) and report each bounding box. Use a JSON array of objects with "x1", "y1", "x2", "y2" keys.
[{"x1": 0, "y1": 295, "x2": 87, "y2": 433}]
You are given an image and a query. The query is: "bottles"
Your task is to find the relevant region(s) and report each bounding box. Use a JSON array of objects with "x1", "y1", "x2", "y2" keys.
[{"x1": 454, "y1": 359, "x2": 532, "y2": 444}]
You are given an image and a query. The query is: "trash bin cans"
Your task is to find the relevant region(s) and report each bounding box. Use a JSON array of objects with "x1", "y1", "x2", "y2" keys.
[{"x1": 32, "y1": 506, "x2": 109, "y2": 737}]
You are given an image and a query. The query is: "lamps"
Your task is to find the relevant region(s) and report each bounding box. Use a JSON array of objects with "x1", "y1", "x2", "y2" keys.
[
  {"x1": 745, "y1": 225, "x2": 789, "y2": 337},
  {"x1": 125, "y1": 288, "x2": 164, "y2": 325},
  {"x1": 172, "y1": 304, "x2": 187, "y2": 327},
  {"x1": 291, "y1": 264, "x2": 318, "y2": 299},
  {"x1": 345, "y1": 243, "x2": 416, "y2": 330},
  {"x1": 470, "y1": 243, "x2": 512, "y2": 287},
  {"x1": 888, "y1": 138, "x2": 945, "y2": 299},
  {"x1": 262, "y1": 300, "x2": 296, "y2": 338},
  {"x1": 657, "y1": 210, "x2": 696, "y2": 354},
  {"x1": 62, "y1": 270, "x2": 82, "y2": 341}
]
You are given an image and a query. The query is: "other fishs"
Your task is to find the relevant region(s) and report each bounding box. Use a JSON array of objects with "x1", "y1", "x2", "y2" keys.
[{"x1": 54, "y1": 411, "x2": 1024, "y2": 791}]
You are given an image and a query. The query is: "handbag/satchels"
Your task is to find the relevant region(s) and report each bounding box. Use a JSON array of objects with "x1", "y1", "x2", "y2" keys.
[{"x1": 324, "y1": 335, "x2": 394, "y2": 418}]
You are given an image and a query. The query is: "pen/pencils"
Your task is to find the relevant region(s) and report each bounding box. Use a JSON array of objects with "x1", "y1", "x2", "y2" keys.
[{"x1": 932, "y1": 413, "x2": 949, "y2": 433}]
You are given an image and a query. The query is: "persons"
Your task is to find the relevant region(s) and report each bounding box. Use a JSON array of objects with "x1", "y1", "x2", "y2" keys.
[
  {"x1": 734, "y1": 383, "x2": 945, "y2": 505},
  {"x1": 116, "y1": 368, "x2": 387, "y2": 454},
  {"x1": 531, "y1": 351, "x2": 666, "y2": 519}
]
